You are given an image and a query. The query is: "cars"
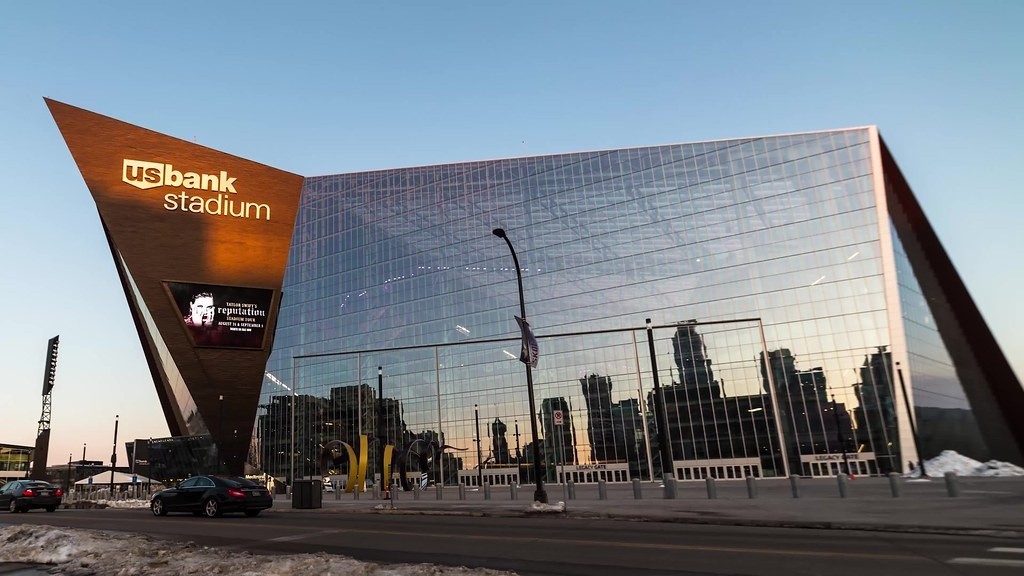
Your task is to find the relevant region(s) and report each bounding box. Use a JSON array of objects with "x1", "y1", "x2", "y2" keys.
[
  {"x1": 0, "y1": 480, "x2": 65, "y2": 514},
  {"x1": 149, "y1": 475, "x2": 274, "y2": 517}
]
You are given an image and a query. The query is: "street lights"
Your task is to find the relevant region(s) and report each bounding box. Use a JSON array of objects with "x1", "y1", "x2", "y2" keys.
[{"x1": 483, "y1": 220, "x2": 553, "y2": 506}]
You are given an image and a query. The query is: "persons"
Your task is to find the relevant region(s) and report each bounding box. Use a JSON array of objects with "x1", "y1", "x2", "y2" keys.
[{"x1": 185, "y1": 291, "x2": 215, "y2": 327}]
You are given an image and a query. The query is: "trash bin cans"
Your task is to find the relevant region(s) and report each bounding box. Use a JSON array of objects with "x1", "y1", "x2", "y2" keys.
[{"x1": 292, "y1": 479, "x2": 322, "y2": 509}]
